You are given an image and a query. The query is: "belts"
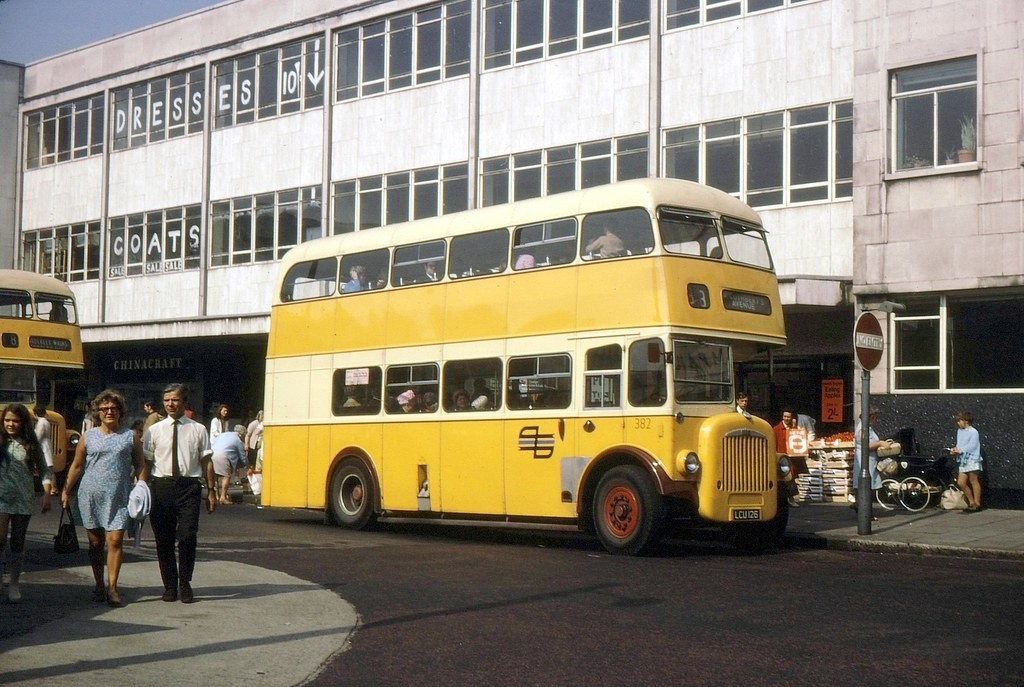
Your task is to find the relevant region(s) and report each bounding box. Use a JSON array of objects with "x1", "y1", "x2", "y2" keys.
[{"x1": 151, "y1": 476, "x2": 198, "y2": 482}]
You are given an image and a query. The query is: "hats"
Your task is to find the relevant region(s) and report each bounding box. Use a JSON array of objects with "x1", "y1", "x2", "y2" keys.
[{"x1": 397, "y1": 390, "x2": 415, "y2": 405}]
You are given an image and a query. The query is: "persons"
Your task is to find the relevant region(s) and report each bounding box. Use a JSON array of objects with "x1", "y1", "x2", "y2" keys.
[
  {"x1": 513, "y1": 236, "x2": 539, "y2": 270},
  {"x1": 585, "y1": 221, "x2": 628, "y2": 260},
  {"x1": 143, "y1": 382, "x2": 216, "y2": 603},
  {"x1": 130, "y1": 400, "x2": 168, "y2": 484},
  {"x1": 60, "y1": 390, "x2": 147, "y2": 607},
  {"x1": 449, "y1": 378, "x2": 498, "y2": 411},
  {"x1": 342, "y1": 264, "x2": 370, "y2": 293},
  {"x1": 849, "y1": 404, "x2": 891, "y2": 521},
  {"x1": 949, "y1": 412, "x2": 983, "y2": 513},
  {"x1": 735, "y1": 391, "x2": 749, "y2": 414},
  {"x1": 393, "y1": 385, "x2": 438, "y2": 413},
  {"x1": 417, "y1": 260, "x2": 443, "y2": 284},
  {"x1": 0, "y1": 403, "x2": 52, "y2": 604},
  {"x1": 210, "y1": 404, "x2": 264, "y2": 504},
  {"x1": 33, "y1": 404, "x2": 54, "y2": 512},
  {"x1": 343, "y1": 382, "x2": 381, "y2": 408},
  {"x1": 509, "y1": 381, "x2": 570, "y2": 409},
  {"x1": 772, "y1": 407, "x2": 808, "y2": 507}
]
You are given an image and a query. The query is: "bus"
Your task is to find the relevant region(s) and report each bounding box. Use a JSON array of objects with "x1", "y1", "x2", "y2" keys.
[
  {"x1": 0, "y1": 267, "x2": 87, "y2": 495},
  {"x1": 255, "y1": 174, "x2": 792, "y2": 559}
]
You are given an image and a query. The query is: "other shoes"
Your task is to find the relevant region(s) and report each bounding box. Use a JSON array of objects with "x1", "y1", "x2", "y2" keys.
[
  {"x1": 50, "y1": 488, "x2": 59, "y2": 496},
  {"x1": 850, "y1": 502, "x2": 858, "y2": 512},
  {"x1": 788, "y1": 497, "x2": 800, "y2": 508},
  {"x1": 162, "y1": 588, "x2": 178, "y2": 601},
  {"x1": 964, "y1": 505, "x2": 984, "y2": 513},
  {"x1": 107, "y1": 587, "x2": 121, "y2": 606},
  {"x1": 219, "y1": 497, "x2": 234, "y2": 505},
  {"x1": 179, "y1": 581, "x2": 193, "y2": 603},
  {"x1": 871, "y1": 515, "x2": 878, "y2": 521},
  {"x1": 92, "y1": 583, "x2": 105, "y2": 601}
]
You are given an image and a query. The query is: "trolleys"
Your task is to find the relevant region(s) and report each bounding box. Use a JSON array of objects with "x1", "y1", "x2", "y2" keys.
[{"x1": 871, "y1": 447, "x2": 952, "y2": 513}]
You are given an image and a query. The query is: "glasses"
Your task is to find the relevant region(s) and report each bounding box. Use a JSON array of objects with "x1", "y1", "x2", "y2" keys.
[{"x1": 98, "y1": 407, "x2": 119, "y2": 413}]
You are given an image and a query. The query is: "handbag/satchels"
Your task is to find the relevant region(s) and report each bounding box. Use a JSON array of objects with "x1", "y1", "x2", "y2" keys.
[
  {"x1": 940, "y1": 484, "x2": 968, "y2": 510},
  {"x1": 53, "y1": 505, "x2": 79, "y2": 554},
  {"x1": 246, "y1": 466, "x2": 262, "y2": 496},
  {"x1": 877, "y1": 439, "x2": 901, "y2": 457}
]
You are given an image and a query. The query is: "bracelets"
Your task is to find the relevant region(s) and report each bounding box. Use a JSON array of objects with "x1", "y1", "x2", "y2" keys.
[{"x1": 208, "y1": 488, "x2": 215, "y2": 491}]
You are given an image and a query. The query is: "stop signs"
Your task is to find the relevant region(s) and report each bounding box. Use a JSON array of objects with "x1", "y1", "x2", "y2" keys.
[{"x1": 852, "y1": 311, "x2": 885, "y2": 372}]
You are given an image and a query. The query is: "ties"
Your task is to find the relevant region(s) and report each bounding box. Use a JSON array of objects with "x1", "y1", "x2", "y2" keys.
[{"x1": 172, "y1": 420, "x2": 179, "y2": 481}]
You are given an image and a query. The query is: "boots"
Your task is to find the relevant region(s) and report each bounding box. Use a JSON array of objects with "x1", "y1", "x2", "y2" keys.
[
  {"x1": 0, "y1": 551, "x2": 5, "y2": 593},
  {"x1": 8, "y1": 551, "x2": 23, "y2": 602}
]
